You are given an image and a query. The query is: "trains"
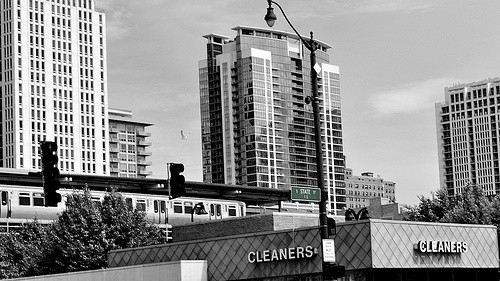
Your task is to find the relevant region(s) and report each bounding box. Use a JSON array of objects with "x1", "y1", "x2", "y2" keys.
[{"x1": 0, "y1": 184, "x2": 247, "y2": 225}]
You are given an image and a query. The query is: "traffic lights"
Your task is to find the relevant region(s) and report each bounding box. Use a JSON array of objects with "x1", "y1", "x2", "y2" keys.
[
  {"x1": 39, "y1": 141, "x2": 62, "y2": 207},
  {"x1": 330, "y1": 266, "x2": 346, "y2": 279},
  {"x1": 166, "y1": 162, "x2": 186, "y2": 200}
]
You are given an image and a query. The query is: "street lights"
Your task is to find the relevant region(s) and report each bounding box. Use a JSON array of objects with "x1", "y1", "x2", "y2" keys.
[{"x1": 264, "y1": 0, "x2": 332, "y2": 281}]
